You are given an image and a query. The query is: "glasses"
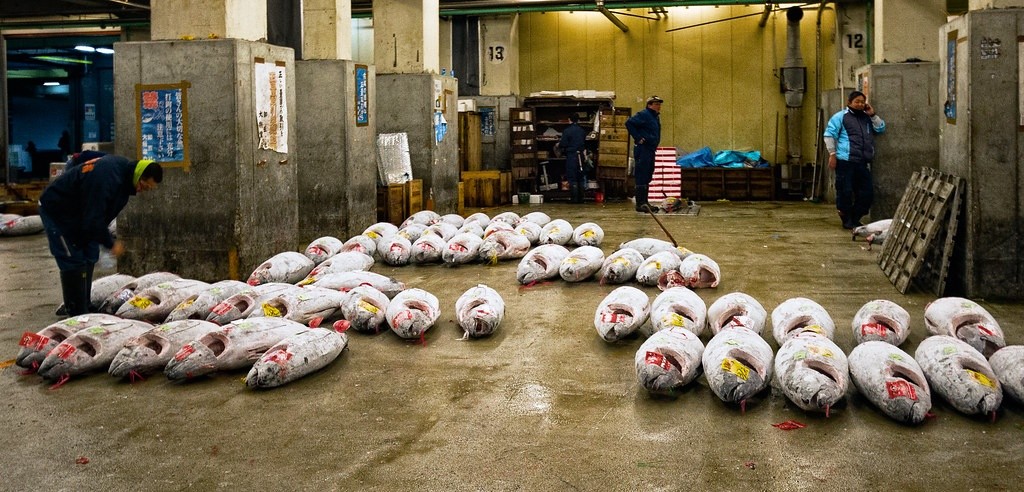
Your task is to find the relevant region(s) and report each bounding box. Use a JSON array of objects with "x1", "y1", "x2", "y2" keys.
[{"x1": 139, "y1": 180, "x2": 159, "y2": 192}]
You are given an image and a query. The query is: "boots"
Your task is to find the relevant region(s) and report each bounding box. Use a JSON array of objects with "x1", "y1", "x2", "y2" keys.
[
  {"x1": 87, "y1": 265, "x2": 98, "y2": 312},
  {"x1": 565, "y1": 183, "x2": 578, "y2": 204},
  {"x1": 578, "y1": 182, "x2": 584, "y2": 204},
  {"x1": 855, "y1": 213, "x2": 865, "y2": 227},
  {"x1": 60, "y1": 271, "x2": 89, "y2": 317},
  {"x1": 636, "y1": 185, "x2": 658, "y2": 213},
  {"x1": 841, "y1": 214, "x2": 856, "y2": 229}
]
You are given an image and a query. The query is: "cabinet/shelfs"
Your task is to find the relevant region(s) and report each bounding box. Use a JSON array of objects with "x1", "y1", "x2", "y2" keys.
[{"x1": 509, "y1": 96, "x2": 633, "y2": 201}]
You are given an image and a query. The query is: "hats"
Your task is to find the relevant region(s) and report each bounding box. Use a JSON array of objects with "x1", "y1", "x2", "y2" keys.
[{"x1": 647, "y1": 96, "x2": 663, "y2": 102}]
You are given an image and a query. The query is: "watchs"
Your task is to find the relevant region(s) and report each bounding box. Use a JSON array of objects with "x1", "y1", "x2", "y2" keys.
[{"x1": 870, "y1": 112, "x2": 876, "y2": 117}]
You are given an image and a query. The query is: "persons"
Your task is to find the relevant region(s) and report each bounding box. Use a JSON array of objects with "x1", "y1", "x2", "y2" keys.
[
  {"x1": 36, "y1": 155, "x2": 164, "y2": 318},
  {"x1": 823, "y1": 91, "x2": 886, "y2": 229},
  {"x1": 26, "y1": 141, "x2": 38, "y2": 167},
  {"x1": 557, "y1": 113, "x2": 586, "y2": 204},
  {"x1": 625, "y1": 95, "x2": 663, "y2": 213},
  {"x1": 58, "y1": 130, "x2": 72, "y2": 161}
]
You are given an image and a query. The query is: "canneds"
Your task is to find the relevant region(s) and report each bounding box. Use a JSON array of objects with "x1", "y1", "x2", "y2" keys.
[{"x1": 484, "y1": 115, "x2": 493, "y2": 135}]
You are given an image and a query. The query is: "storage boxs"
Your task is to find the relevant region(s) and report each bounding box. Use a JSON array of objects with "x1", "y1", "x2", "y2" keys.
[
  {"x1": 648, "y1": 148, "x2": 681, "y2": 202},
  {"x1": 529, "y1": 194, "x2": 544, "y2": 205},
  {"x1": 458, "y1": 98, "x2": 476, "y2": 112}
]
